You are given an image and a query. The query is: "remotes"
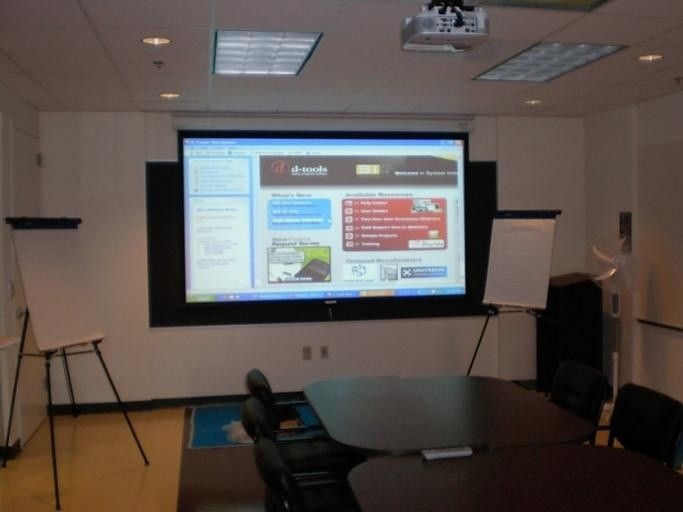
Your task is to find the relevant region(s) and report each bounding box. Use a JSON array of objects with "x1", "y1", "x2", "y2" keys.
[{"x1": 421, "y1": 446, "x2": 474, "y2": 461}]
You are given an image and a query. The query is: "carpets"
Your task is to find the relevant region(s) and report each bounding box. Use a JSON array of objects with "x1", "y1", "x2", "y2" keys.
[{"x1": 184, "y1": 397, "x2": 326, "y2": 452}]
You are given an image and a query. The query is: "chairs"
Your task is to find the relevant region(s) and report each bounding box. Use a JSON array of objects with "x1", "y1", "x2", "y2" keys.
[
  {"x1": 236, "y1": 367, "x2": 369, "y2": 511},
  {"x1": 605, "y1": 383, "x2": 683, "y2": 473},
  {"x1": 546, "y1": 360, "x2": 610, "y2": 432}
]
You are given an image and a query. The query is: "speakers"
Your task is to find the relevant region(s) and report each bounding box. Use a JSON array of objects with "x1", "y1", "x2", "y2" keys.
[{"x1": 536, "y1": 271, "x2": 604, "y2": 397}]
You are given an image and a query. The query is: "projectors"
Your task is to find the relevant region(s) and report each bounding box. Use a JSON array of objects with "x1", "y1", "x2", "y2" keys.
[{"x1": 400, "y1": 4, "x2": 490, "y2": 53}]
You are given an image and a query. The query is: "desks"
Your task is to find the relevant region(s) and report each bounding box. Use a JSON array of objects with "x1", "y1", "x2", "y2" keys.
[
  {"x1": 304, "y1": 374, "x2": 597, "y2": 453},
  {"x1": 349, "y1": 442, "x2": 683, "y2": 511}
]
